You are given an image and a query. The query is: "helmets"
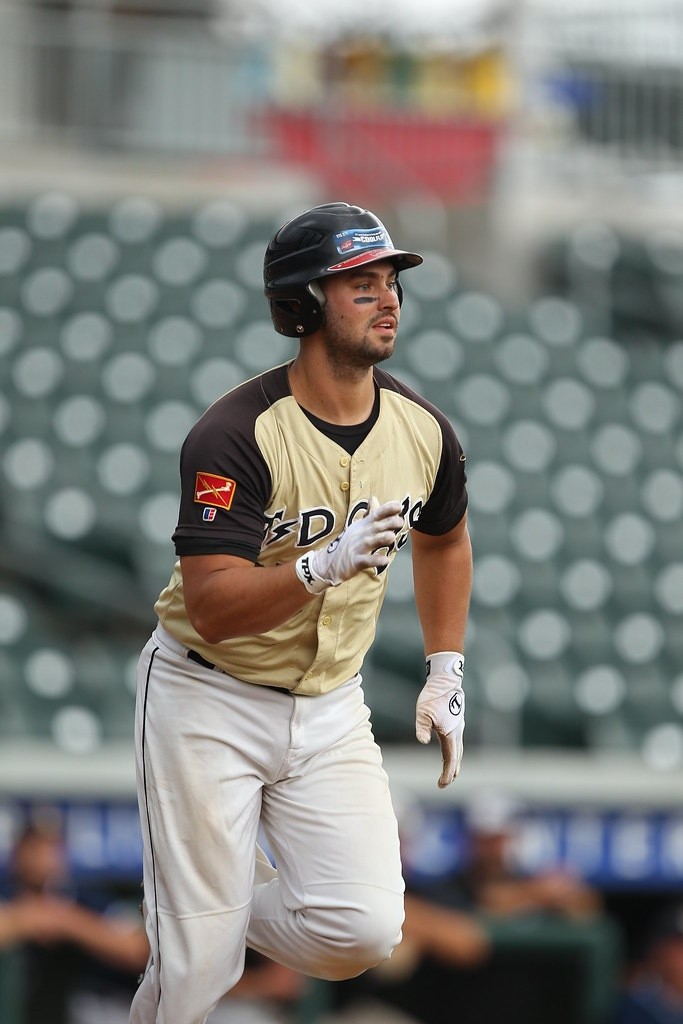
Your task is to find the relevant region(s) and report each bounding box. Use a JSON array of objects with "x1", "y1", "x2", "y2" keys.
[{"x1": 264, "y1": 201, "x2": 424, "y2": 339}]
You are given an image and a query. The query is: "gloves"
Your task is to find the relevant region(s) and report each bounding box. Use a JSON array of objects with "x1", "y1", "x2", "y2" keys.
[
  {"x1": 295, "y1": 497, "x2": 405, "y2": 595},
  {"x1": 415, "y1": 652, "x2": 466, "y2": 789}
]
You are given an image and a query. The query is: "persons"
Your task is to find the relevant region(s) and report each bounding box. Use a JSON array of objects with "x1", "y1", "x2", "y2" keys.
[
  {"x1": 1, "y1": 807, "x2": 679, "y2": 1024},
  {"x1": 113, "y1": 202, "x2": 476, "y2": 1024}
]
